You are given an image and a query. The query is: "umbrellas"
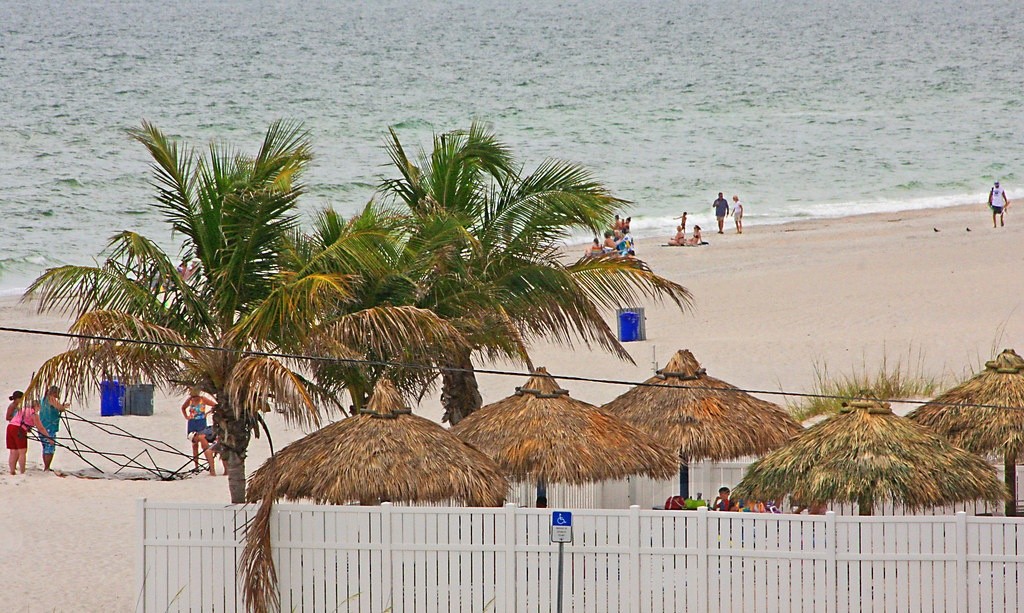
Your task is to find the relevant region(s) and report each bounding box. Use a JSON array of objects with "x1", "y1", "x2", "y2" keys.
[
  {"x1": 727, "y1": 402, "x2": 1015, "y2": 516},
  {"x1": 901, "y1": 349, "x2": 1024, "y2": 516},
  {"x1": 243, "y1": 349, "x2": 809, "y2": 508}
]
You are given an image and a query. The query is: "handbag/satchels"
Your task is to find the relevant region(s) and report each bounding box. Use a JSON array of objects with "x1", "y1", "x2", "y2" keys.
[
  {"x1": 684, "y1": 499, "x2": 706, "y2": 509},
  {"x1": 750, "y1": 501, "x2": 765, "y2": 512},
  {"x1": 665, "y1": 495, "x2": 686, "y2": 510}
]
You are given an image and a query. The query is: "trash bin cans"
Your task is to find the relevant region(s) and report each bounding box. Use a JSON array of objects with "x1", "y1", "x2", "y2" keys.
[
  {"x1": 619, "y1": 312, "x2": 639, "y2": 342},
  {"x1": 99, "y1": 379, "x2": 126, "y2": 417},
  {"x1": 128, "y1": 384, "x2": 156, "y2": 416}
]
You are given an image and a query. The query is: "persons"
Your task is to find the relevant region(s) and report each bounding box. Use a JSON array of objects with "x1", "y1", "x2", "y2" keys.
[
  {"x1": 731, "y1": 195, "x2": 743, "y2": 234},
  {"x1": 673, "y1": 212, "x2": 688, "y2": 233},
  {"x1": 712, "y1": 487, "x2": 828, "y2": 515},
  {"x1": 713, "y1": 193, "x2": 729, "y2": 234},
  {"x1": 585, "y1": 215, "x2": 635, "y2": 257},
  {"x1": 176, "y1": 261, "x2": 196, "y2": 281},
  {"x1": 6, "y1": 385, "x2": 228, "y2": 475},
  {"x1": 668, "y1": 225, "x2": 702, "y2": 246},
  {"x1": 988, "y1": 182, "x2": 1008, "y2": 227}
]
